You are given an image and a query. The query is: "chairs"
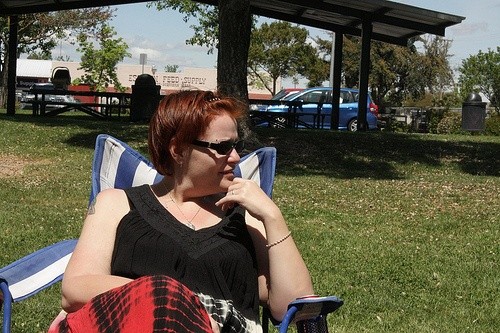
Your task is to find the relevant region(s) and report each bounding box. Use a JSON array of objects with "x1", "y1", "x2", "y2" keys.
[{"x1": 0, "y1": 135, "x2": 343, "y2": 333}]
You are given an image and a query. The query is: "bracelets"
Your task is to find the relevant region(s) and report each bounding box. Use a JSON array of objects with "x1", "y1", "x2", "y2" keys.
[{"x1": 266, "y1": 231, "x2": 292, "y2": 247}]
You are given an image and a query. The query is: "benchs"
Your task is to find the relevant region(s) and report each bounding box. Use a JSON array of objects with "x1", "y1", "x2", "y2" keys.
[
  {"x1": 252, "y1": 109, "x2": 330, "y2": 130},
  {"x1": 22, "y1": 100, "x2": 130, "y2": 121}
]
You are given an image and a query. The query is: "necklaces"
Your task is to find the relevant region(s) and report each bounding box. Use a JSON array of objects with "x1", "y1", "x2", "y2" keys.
[{"x1": 162, "y1": 179, "x2": 204, "y2": 231}]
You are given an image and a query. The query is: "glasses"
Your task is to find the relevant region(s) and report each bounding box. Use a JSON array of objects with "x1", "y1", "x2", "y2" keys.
[{"x1": 191, "y1": 136, "x2": 246, "y2": 155}]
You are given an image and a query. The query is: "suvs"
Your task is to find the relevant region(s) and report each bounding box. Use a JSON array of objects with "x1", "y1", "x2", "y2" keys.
[{"x1": 249, "y1": 86, "x2": 379, "y2": 134}]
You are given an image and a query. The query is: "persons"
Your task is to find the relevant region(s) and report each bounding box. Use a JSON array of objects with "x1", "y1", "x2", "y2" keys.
[{"x1": 48, "y1": 90, "x2": 314, "y2": 333}]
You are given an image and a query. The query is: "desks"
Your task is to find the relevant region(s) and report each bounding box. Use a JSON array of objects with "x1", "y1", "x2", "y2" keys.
[
  {"x1": 250, "y1": 98, "x2": 302, "y2": 130},
  {"x1": 28, "y1": 89, "x2": 132, "y2": 117}
]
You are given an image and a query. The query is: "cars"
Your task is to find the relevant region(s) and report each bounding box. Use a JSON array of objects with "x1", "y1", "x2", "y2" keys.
[{"x1": 20, "y1": 82, "x2": 67, "y2": 112}]
